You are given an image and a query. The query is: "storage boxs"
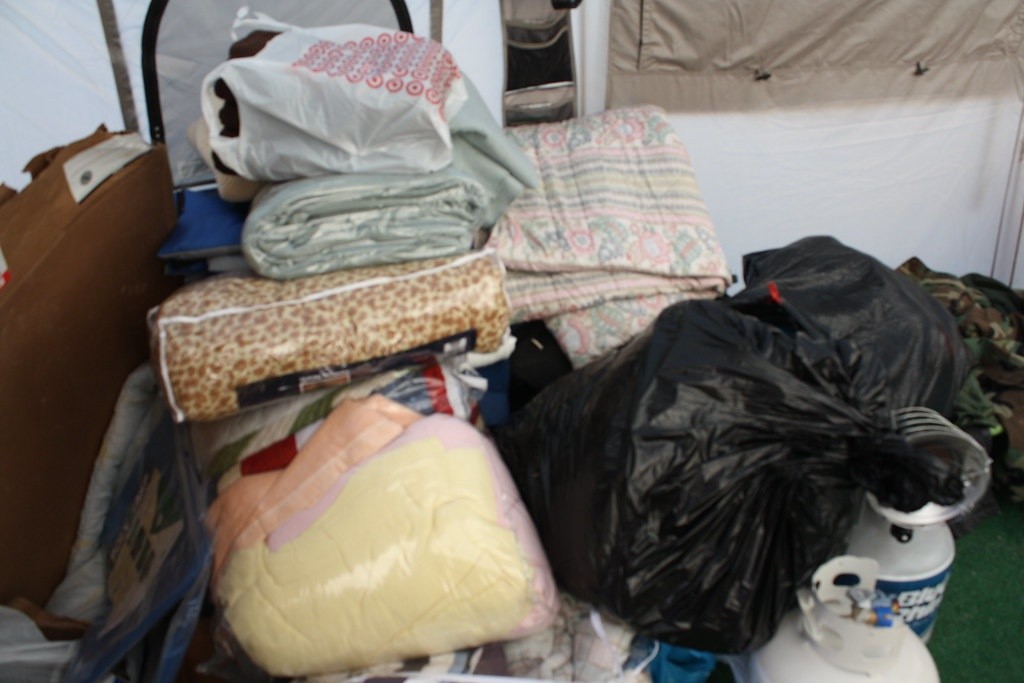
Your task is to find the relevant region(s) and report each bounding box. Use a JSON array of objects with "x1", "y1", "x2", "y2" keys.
[{"x1": 0, "y1": 124, "x2": 177, "y2": 613}]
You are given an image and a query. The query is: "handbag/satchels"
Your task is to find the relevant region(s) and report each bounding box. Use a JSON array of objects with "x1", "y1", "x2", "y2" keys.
[{"x1": 200, "y1": 7, "x2": 469, "y2": 183}]
